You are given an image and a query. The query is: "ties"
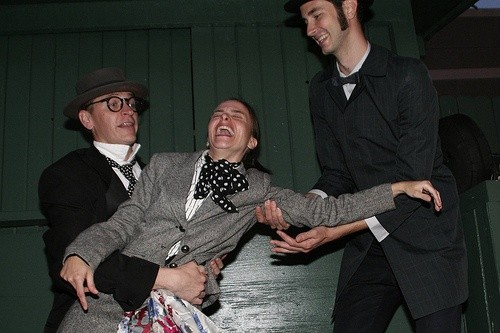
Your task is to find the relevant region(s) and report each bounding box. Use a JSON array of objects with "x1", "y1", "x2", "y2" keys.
[{"x1": 101, "y1": 150, "x2": 140, "y2": 198}]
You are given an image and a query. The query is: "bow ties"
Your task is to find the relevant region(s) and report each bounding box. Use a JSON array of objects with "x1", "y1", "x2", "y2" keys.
[
  {"x1": 332, "y1": 72, "x2": 358, "y2": 89},
  {"x1": 193, "y1": 153, "x2": 250, "y2": 214}
]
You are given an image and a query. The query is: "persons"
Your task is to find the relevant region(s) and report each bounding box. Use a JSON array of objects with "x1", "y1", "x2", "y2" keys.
[
  {"x1": 256, "y1": 0, "x2": 469, "y2": 333},
  {"x1": 38, "y1": 69, "x2": 228, "y2": 333},
  {"x1": 57, "y1": 97, "x2": 442, "y2": 333}
]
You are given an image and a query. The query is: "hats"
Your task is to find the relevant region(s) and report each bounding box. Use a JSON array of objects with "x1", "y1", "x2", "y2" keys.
[
  {"x1": 284, "y1": 0, "x2": 310, "y2": 12},
  {"x1": 63, "y1": 66, "x2": 150, "y2": 121}
]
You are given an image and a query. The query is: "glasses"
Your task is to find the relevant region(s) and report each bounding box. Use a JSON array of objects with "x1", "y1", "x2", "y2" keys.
[{"x1": 80, "y1": 96, "x2": 139, "y2": 115}]
